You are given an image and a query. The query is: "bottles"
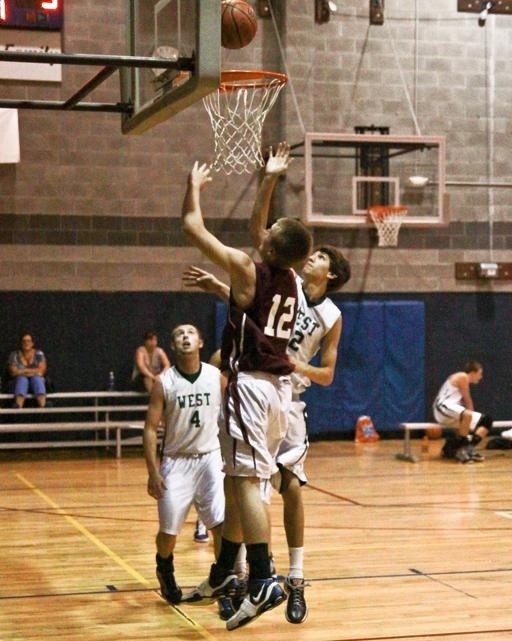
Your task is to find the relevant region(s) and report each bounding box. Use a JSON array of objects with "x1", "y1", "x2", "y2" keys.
[
  {"x1": 107, "y1": 371, "x2": 115, "y2": 391},
  {"x1": 421, "y1": 436, "x2": 431, "y2": 456}
]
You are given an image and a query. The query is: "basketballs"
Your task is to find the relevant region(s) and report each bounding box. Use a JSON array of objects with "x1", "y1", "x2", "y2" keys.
[
  {"x1": 221, "y1": 0, "x2": 258, "y2": 49},
  {"x1": 427, "y1": 428, "x2": 443, "y2": 439}
]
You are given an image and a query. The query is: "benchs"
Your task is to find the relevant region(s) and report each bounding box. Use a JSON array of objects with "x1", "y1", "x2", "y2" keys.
[
  {"x1": 396, "y1": 420, "x2": 512, "y2": 463},
  {"x1": 0, "y1": 391, "x2": 164, "y2": 458}
]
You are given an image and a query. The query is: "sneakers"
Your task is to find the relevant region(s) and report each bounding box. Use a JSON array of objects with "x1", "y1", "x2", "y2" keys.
[
  {"x1": 156, "y1": 566, "x2": 183, "y2": 605},
  {"x1": 181, "y1": 553, "x2": 311, "y2": 632},
  {"x1": 194, "y1": 518, "x2": 210, "y2": 544},
  {"x1": 469, "y1": 449, "x2": 484, "y2": 462},
  {"x1": 455, "y1": 448, "x2": 474, "y2": 465}
]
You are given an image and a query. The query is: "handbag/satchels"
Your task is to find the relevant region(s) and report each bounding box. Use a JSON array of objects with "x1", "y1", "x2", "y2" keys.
[
  {"x1": 46, "y1": 375, "x2": 55, "y2": 393},
  {"x1": 119, "y1": 372, "x2": 143, "y2": 392}
]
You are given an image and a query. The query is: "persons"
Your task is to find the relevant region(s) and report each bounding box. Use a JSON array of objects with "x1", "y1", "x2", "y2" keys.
[
  {"x1": 145, "y1": 325, "x2": 229, "y2": 606},
  {"x1": 434, "y1": 360, "x2": 492, "y2": 466},
  {"x1": 193, "y1": 513, "x2": 211, "y2": 543},
  {"x1": 131, "y1": 332, "x2": 172, "y2": 396},
  {"x1": 5, "y1": 330, "x2": 50, "y2": 412},
  {"x1": 185, "y1": 243, "x2": 351, "y2": 626},
  {"x1": 182, "y1": 140, "x2": 312, "y2": 633}
]
